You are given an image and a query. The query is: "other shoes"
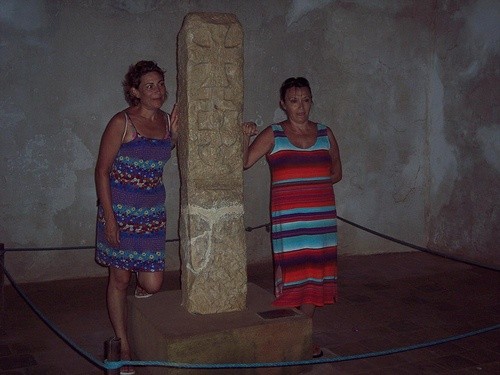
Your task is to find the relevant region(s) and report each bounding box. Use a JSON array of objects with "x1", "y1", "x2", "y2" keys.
[{"x1": 312, "y1": 343, "x2": 323, "y2": 358}]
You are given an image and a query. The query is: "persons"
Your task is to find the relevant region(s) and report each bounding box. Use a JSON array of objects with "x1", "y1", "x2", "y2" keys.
[
  {"x1": 95, "y1": 60, "x2": 179, "y2": 375},
  {"x1": 244, "y1": 77, "x2": 342, "y2": 358}
]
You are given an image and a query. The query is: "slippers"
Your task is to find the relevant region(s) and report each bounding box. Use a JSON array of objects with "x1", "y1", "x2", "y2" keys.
[
  {"x1": 120, "y1": 357, "x2": 135, "y2": 375},
  {"x1": 135, "y1": 272, "x2": 153, "y2": 298}
]
